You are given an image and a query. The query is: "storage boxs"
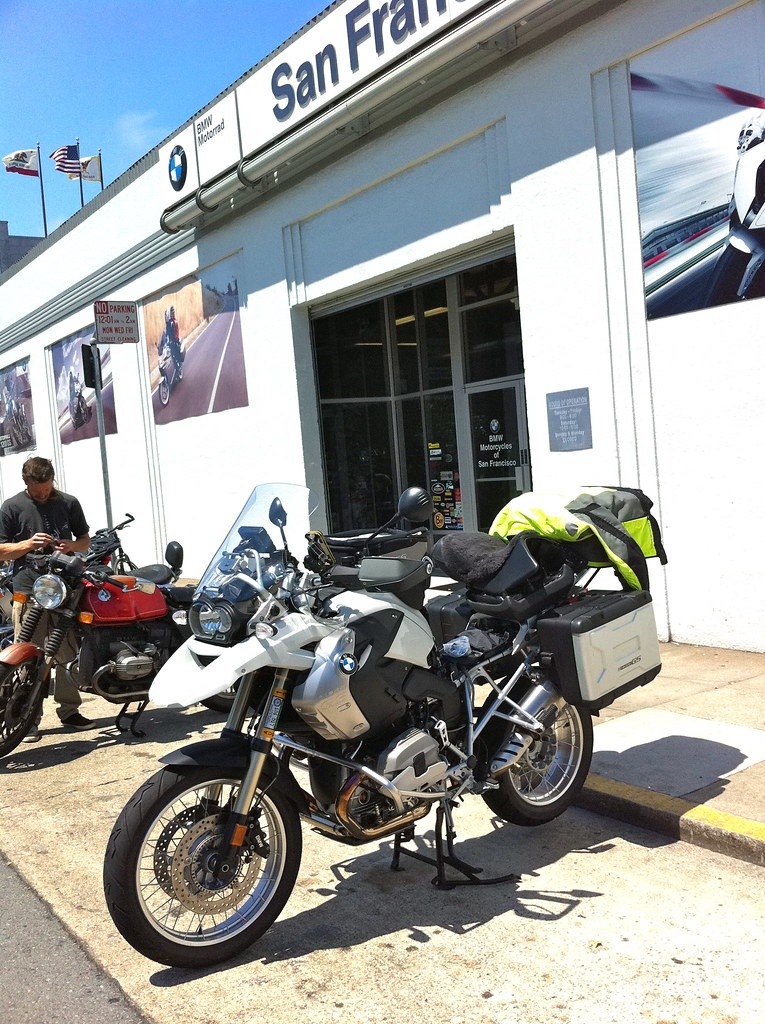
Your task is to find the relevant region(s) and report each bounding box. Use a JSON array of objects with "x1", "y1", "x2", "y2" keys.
[{"x1": 537, "y1": 590, "x2": 662, "y2": 711}]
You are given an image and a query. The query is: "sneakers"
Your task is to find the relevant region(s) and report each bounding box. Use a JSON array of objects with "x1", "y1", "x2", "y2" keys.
[
  {"x1": 60, "y1": 712, "x2": 96, "y2": 729},
  {"x1": 22, "y1": 724, "x2": 40, "y2": 742}
]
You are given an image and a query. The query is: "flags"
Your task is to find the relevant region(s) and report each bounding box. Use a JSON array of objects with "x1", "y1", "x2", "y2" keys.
[
  {"x1": 67, "y1": 153, "x2": 101, "y2": 182},
  {"x1": 2, "y1": 150, "x2": 39, "y2": 178},
  {"x1": 48, "y1": 142, "x2": 80, "y2": 173}
]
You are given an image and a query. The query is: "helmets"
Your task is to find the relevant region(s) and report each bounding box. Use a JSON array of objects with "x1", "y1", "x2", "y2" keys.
[
  {"x1": 3, "y1": 373, "x2": 13, "y2": 382},
  {"x1": 164, "y1": 306, "x2": 176, "y2": 323}
]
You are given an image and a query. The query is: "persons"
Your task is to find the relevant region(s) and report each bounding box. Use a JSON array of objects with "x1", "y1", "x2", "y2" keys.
[
  {"x1": 160, "y1": 305, "x2": 182, "y2": 371},
  {"x1": 69, "y1": 371, "x2": 84, "y2": 415},
  {"x1": 2, "y1": 375, "x2": 18, "y2": 430},
  {"x1": 0, "y1": 457, "x2": 95, "y2": 742}
]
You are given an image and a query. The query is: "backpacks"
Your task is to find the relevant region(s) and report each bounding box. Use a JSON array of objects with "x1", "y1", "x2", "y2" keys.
[{"x1": 489, "y1": 486, "x2": 666, "y2": 589}]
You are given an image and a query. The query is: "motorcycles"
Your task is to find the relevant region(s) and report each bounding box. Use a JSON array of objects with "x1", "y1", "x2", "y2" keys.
[
  {"x1": 102, "y1": 484, "x2": 667, "y2": 969},
  {"x1": 69, "y1": 384, "x2": 90, "y2": 430},
  {"x1": 2, "y1": 397, "x2": 33, "y2": 448},
  {"x1": 0, "y1": 514, "x2": 256, "y2": 759},
  {"x1": 690, "y1": 111, "x2": 765, "y2": 311},
  {"x1": 154, "y1": 339, "x2": 186, "y2": 405}
]
